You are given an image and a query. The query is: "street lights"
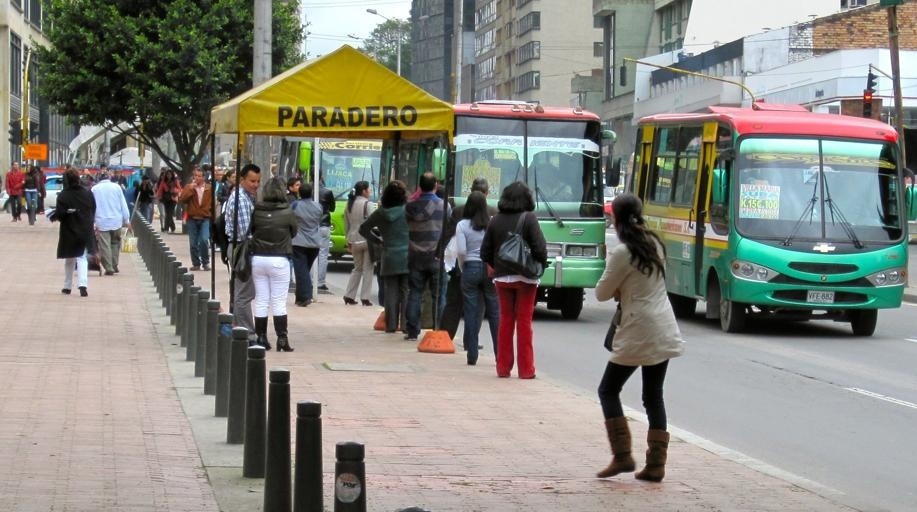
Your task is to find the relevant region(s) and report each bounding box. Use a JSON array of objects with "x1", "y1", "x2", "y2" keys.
[{"x1": 365, "y1": 6, "x2": 428, "y2": 76}]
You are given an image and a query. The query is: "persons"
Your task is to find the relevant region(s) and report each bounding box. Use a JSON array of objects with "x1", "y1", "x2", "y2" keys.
[
  {"x1": 6, "y1": 161, "x2": 238, "y2": 297},
  {"x1": 311, "y1": 171, "x2": 335, "y2": 290},
  {"x1": 343, "y1": 170, "x2": 501, "y2": 365},
  {"x1": 290, "y1": 184, "x2": 323, "y2": 305},
  {"x1": 227, "y1": 164, "x2": 261, "y2": 347},
  {"x1": 248, "y1": 176, "x2": 298, "y2": 352},
  {"x1": 284, "y1": 178, "x2": 301, "y2": 204},
  {"x1": 596, "y1": 193, "x2": 687, "y2": 481},
  {"x1": 480, "y1": 182, "x2": 547, "y2": 378}
]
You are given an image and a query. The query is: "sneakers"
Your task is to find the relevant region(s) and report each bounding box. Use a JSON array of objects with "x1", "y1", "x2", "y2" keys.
[
  {"x1": 61, "y1": 289, "x2": 71, "y2": 294},
  {"x1": 189, "y1": 263, "x2": 211, "y2": 271},
  {"x1": 10, "y1": 210, "x2": 47, "y2": 226},
  {"x1": 295, "y1": 299, "x2": 313, "y2": 307},
  {"x1": 78, "y1": 285, "x2": 88, "y2": 296},
  {"x1": 403, "y1": 334, "x2": 418, "y2": 341},
  {"x1": 316, "y1": 284, "x2": 329, "y2": 290},
  {"x1": 102, "y1": 266, "x2": 121, "y2": 274}
]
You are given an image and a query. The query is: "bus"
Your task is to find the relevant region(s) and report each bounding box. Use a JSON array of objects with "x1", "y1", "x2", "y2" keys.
[
  {"x1": 271, "y1": 134, "x2": 383, "y2": 271},
  {"x1": 377, "y1": 97, "x2": 624, "y2": 323},
  {"x1": 621, "y1": 96, "x2": 917, "y2": 340}
]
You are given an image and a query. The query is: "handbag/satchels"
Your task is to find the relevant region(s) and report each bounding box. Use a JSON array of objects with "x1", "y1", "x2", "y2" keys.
[
  {"x1": 603, "y1": 303, "x2": 622, "y2": 352},
  {"x1": 231, "y1": 208, "x2": 256, "y2": 283},
  {"x1": 495, "y1": 212, "x2": 544, "y2": 278}
]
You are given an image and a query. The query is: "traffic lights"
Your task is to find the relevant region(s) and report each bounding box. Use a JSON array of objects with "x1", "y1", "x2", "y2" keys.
[
  {"x1": 6, "y1": 119, "x2": 22, "y2": 146},
  {"x1": 30, "y1": 118, "x2": 39, "y2": 142},
  {"x1": 866, "y1": 72, "x2": 878, "y2": 94},
  {"x1": 863, "y1": 89, "x2": 874, "y2": 117}
]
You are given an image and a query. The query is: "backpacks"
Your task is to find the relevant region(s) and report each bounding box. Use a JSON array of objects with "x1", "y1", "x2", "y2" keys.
[{"x1": 210, "y1": 198, "x2": 231, "y2": 274}]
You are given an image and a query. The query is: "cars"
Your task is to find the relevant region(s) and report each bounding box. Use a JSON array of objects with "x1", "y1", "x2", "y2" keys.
[
  {"x1": 0, "y1": 174, "x2": 64, "y2": 216},
  {"x1": 596, "y1": 151, "x2": 636, "y2": 230}
]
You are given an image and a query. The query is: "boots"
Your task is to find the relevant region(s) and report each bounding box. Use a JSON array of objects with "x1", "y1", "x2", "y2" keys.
[
  {"x1": 634, "y1": 427, "x2": 670, "y2": 482},
  {"x1": 273, "y1": 314, "x2": 295, "y2": 352},
  {"x1": 595, "y1": 415, "x2": 636, "y2": 478},
  {"x1": 254, "y1": 315, "x2": 272, "y2": 351}
]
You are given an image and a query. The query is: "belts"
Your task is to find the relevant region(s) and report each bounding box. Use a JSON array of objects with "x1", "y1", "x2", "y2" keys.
[{"x1": 465, "y1": 261, "x2": 487, "y2": 268}]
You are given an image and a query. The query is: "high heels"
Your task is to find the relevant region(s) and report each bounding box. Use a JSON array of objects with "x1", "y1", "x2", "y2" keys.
[
  {"x1": 361, "y1": 298, "x2": 373, "y2": 306},
  {"x1": 343, "y1": 296, "x2": 358, "y2": 305}
]
